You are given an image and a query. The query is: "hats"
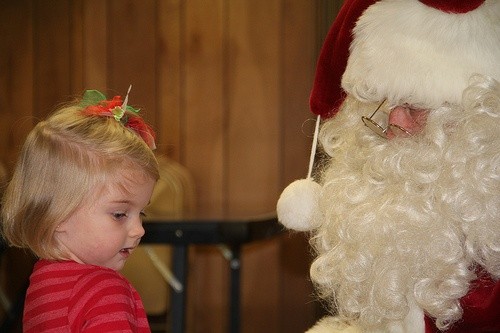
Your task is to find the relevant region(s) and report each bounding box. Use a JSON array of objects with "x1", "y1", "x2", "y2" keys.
[{"x1": 270, "y1": 0, "x2": 500, "y2": 235}]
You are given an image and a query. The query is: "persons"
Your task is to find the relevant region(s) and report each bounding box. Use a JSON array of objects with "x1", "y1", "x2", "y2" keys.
[
  {"x1": 275, "y1": 1, "x2": 500, "y2": 333},
  {"x1": 0, "y1": 80, "x2": 164, "y2": 333}
]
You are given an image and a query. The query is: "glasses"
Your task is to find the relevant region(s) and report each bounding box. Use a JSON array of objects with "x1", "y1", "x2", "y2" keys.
[{"x1": 359, "y1": 95, "x2": 428, "y2": 145}]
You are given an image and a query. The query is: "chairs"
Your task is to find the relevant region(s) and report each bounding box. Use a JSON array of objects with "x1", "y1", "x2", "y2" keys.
[{"x1": 140, "y1": 212, "x2": 294, "y2": 333}]
